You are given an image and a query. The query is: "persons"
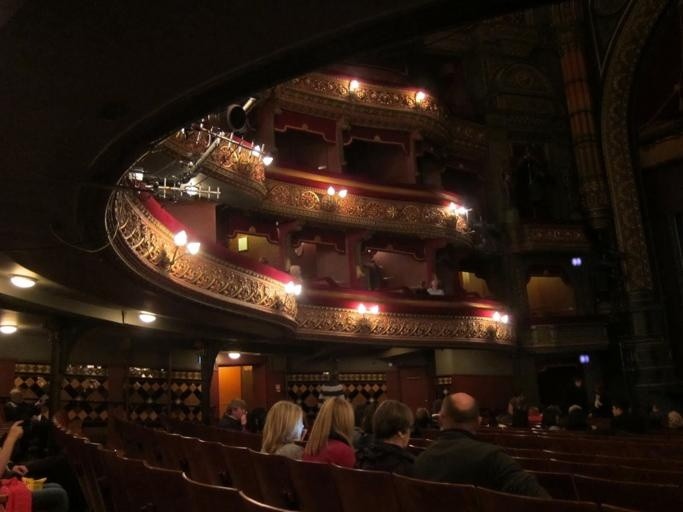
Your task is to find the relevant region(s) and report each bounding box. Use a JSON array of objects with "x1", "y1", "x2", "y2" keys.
[
  {"x1": 221, "y1": 399, "x2": 249, "y2": 431},
  {"x1": 425, "y1": 279, "x2": 445, "y2": 296},
  {"x1": 3, "y1": 387, "x2": 50, "y2": 456},
  {"x1": 301, "y1": 397, "x2": 321, "y2": 431},
  {"x1": 259, "y1": 400, "x2": 304, "y2": 460},
  {"x1": 0, "y1": 451, "x2": 67, "y2": 479},
  {"x1": 300, "y1": 394, "x2": 357, "y2": 469},
  {"x1": 415, "y1": 396, "x2": 682, "y2": 437},
  {"x1": 410, "y1": 278, "x2": 430, "y2": 295},
  {"x1": 289, "y1": 265, "x2": 302, "y2": 278},
  {"x1": 411, "y1": 392, "x2": 553, "y2": 499},
  {"x1": 358, "y1": 401, "x2": 382, "y2": 440},
  {"x1": 253, "y1": 406, "x2": 266, "y2": 428},
  {"x1": 353, "y1": 399, "x2": 416, "y2": 478},
  {"x1": 0, "y1": 419, "x2": 68, "y2": 511}
]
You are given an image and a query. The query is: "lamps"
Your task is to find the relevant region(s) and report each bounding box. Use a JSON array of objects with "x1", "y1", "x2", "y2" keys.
[
  {"x1": 5, "y1": 272, "x2": 39, "y2": 291},
  {"x1": 138, "y1": 310, "x2": 157, "y2": 325},
  {"x1": 227, "y1": 352, "x2": 242, "y2": 359},
  {"x1": 0, "y1": 324, "x2": 18, "y2": 336}
]
